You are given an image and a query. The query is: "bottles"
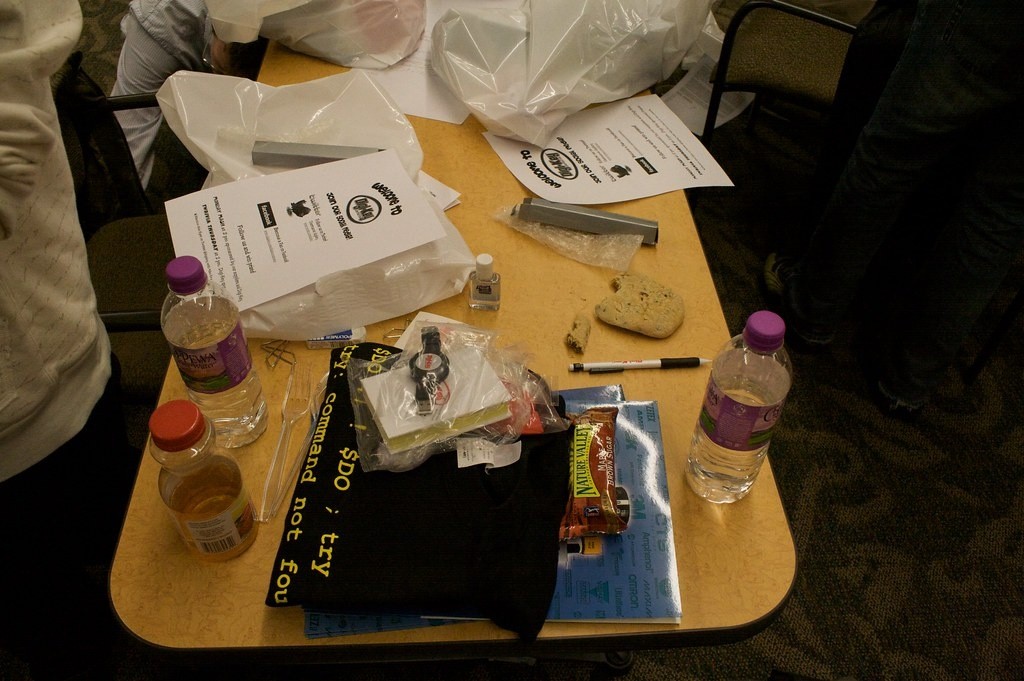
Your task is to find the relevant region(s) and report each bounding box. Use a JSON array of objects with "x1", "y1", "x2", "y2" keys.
[
  {"x1": 148, "y1": 400, "x2": 257, "y2": 564},
  {"x1": 160, "y1": 255, "x2": 269, "y2": 448},
  {"x1": 685, "y1": 311, "x2": 791, "y2": 503}
]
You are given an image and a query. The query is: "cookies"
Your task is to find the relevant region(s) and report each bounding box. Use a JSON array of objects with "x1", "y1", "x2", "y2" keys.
[
  {"x1": 566, "y1": 311, "x2": 591, "y2": 354},
  {"x1": 594, "y1": 271, "x2": 686, "y2": 339}
]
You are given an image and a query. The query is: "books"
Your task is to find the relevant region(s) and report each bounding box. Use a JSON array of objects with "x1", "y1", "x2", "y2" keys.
[
  {"x1": 302, "y1": 384, "x2": 682, "y2": 641},
  {"x1": 360, "y1": 345, "x2": 513, "y2": 455}
]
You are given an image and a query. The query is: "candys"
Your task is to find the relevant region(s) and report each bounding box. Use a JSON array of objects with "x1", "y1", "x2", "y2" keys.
[{"x1": 558, "y1": 407, "x2": 628, "y2": 540}]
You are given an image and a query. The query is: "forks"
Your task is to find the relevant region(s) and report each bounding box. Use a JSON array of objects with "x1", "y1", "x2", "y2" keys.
[{"x1": 258, "y1": 358, "x2": 313, "y2": 524}]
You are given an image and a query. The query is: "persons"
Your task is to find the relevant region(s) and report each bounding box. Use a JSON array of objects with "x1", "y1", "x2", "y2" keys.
[
  {"x1": 110, "y1": 0, "x2": 232, "y2": 192},
  {"x1": 0, "y1": 0, "x2": 130, "y2": 681},
  {"x1": 760, "y1": 0, "x2": 1024, "y2": 423}
]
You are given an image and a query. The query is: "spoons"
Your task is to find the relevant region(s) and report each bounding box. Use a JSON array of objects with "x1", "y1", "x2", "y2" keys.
[{"x1": 271, "y1": 372, "x2": 329, "y2": 519}]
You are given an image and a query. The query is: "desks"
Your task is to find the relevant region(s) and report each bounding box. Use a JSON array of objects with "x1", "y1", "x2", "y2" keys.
[{"x1": 108, "y1": 38, "x2": 797, "y2": 650}]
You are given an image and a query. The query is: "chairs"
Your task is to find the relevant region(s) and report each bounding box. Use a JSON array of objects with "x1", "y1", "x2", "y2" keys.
[
  {"x1": 54, "y1": 61, "x2": 201, "y2": 446},
  {"x1": 689, "y1": 0, "x2": 919, "y2": 268}
]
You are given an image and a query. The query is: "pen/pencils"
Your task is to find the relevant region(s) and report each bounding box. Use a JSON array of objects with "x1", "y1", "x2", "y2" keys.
[{"x1": 563, "y1": 354, "x2": 713, "y2": 378}]
